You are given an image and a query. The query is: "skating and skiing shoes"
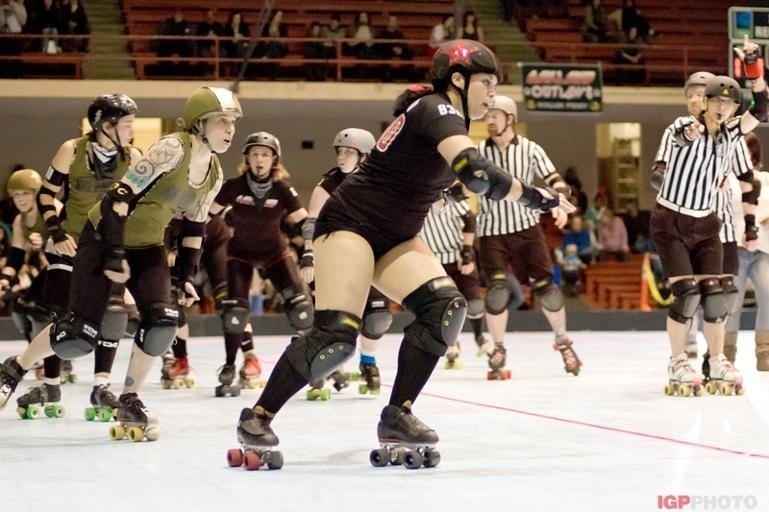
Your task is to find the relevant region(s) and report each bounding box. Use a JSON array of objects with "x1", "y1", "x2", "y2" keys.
[
  {"x1": 0, "y1": 359, "x2": 77, "y2": 419},
  {"x1": 216, "y1": 350, "x2": 284, "y2": 470},
  {"x1": 84, "y1": 354, "x2": 194, "y2": 441},
  {"x1": 445, "y1": 336, "x2": 512, "y2": 380},
  {"x1": 664, "y1": 331, "x2": 769, "y2": 396},
  {"x1": 554, "y1": 339, "x2": 582, "y2": 375}
]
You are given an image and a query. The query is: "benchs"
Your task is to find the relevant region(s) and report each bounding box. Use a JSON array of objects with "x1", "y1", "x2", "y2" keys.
[{"x1": 583, "y1": 252, "x2": 645, "y2": 309}]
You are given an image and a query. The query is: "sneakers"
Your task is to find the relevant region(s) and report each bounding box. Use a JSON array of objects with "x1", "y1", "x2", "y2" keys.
[
  {"x1": 307, "y1": 365, "x2": 380, "y2": 400},
  {"x1": 370, "y1": 400, "x2": 440, "y2": 469}
]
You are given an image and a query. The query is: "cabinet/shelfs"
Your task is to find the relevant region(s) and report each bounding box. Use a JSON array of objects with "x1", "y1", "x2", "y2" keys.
[{"x1": 600, "y1": 154, "x2": 638, "y2": 213}]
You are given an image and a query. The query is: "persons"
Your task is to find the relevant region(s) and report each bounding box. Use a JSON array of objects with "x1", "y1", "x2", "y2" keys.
[
  {"x1": 1, "y1": 83, "x2": 245, "y2": 427},
  {"x1": 1, "y1": 2, "x2": 769, "y2": 388},
  {"x1": 237, "y1": 40, "x2": 577, "y2": 448},
  {"x1": 15, "y1": 90, "x2": 144, "y2": 409}
]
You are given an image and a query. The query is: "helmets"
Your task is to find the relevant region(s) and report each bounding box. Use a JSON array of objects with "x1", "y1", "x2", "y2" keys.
[
  {"x1": 88, "y1": 87, "x2": 281, "y2": 166},
  {"x1": 683, "y1": 71, "x2": 743, "y2": 110},
  {"x1": 332, "y1": 39, "x2": 518, "y2": 153},
  {"x1": 6, "y1": 168, "x2": 42, "y2": 195}
]
